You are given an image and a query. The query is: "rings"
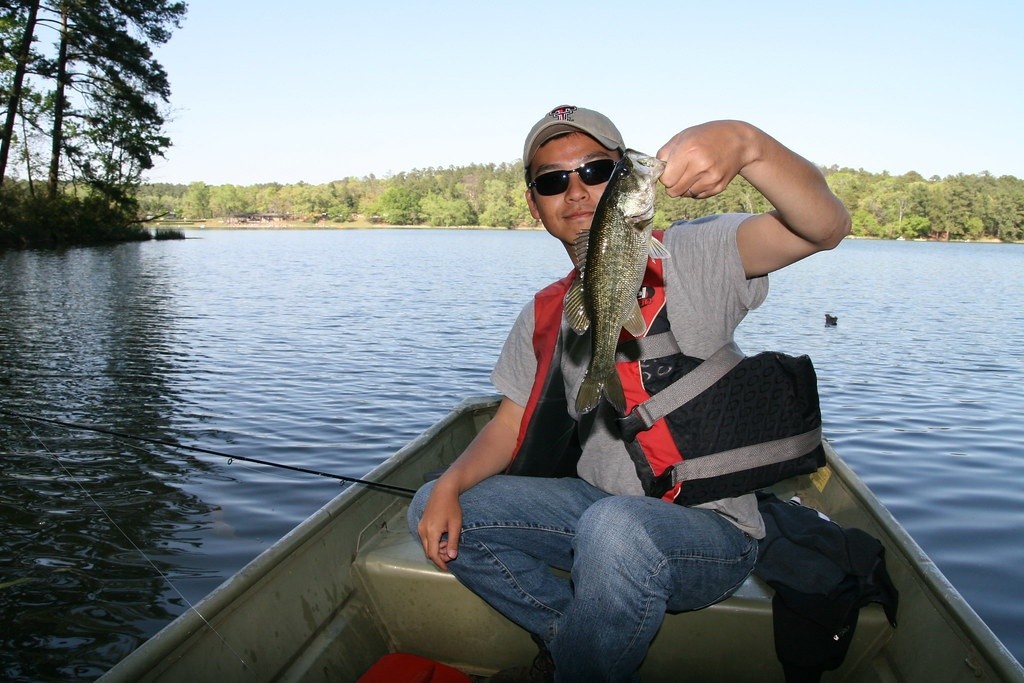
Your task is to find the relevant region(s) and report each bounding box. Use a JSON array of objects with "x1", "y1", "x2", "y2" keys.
[{"x1": 688, "y1": 188, "x2": 698, "y2": 197}]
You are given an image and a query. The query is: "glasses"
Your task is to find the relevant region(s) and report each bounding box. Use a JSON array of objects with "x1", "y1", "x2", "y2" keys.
[{"x1": 528, "y1": 159, "x2": 617, "y2": 196}]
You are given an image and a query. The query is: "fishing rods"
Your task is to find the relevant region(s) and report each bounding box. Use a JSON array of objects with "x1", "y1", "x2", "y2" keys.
[{"x1": 0, "y1": 409, "x2": 418, "y2": 494}]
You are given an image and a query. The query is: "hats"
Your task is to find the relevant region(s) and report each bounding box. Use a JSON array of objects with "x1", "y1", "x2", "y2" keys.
[{"x1": 523, "y1": 104, "x2": 626, "y2": 169}]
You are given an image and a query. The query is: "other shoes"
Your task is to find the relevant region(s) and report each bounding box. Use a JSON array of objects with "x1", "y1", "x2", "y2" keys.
[{"x1": 489, "y1": 632, "x2": 556, "y2": 683}]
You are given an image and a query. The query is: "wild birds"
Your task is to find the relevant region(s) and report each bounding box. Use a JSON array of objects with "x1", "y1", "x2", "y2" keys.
[{"x1": 825, "y1": 313, "x2": 837, "y2": 328}]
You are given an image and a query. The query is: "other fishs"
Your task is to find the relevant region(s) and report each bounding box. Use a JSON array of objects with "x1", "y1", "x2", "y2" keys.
[{"x1": 563, "y1": 147, "x2": 666, "y2": 417}]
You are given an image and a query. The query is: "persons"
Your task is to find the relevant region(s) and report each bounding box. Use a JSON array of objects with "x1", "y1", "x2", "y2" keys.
[{"x1": 406, "y1": 105, "x2": 851, "y2": 683}]
